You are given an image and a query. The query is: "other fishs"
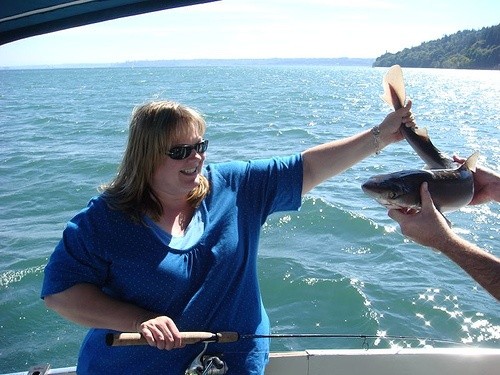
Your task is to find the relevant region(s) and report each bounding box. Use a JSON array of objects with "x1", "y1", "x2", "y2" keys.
[{"x1": 360, "y1": 64, "x2": 480, "y2": 255}]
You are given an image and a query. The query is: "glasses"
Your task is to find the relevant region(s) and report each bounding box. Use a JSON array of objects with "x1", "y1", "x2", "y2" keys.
[{"x1": 158, "y1": 139, "x2": 208, "y2": 160}]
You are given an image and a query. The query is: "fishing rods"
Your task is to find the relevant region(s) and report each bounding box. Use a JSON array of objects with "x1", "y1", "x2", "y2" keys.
[{"x1": 105, "y1": 331, "x2": 462, "y2": 348}]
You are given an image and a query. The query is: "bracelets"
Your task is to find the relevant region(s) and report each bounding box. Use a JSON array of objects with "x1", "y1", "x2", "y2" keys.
[{"x1": 370, "y1": 124, "x2": 382, "y2": 159}]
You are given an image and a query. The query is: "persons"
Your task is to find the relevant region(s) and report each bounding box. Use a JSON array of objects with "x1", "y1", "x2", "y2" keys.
[
  {"x1": 39, "y1": 98, "x2": 417, "y2": 374},
  {"x1": 387, "y1": 155, "x2": 500, "y2": 302}
]
